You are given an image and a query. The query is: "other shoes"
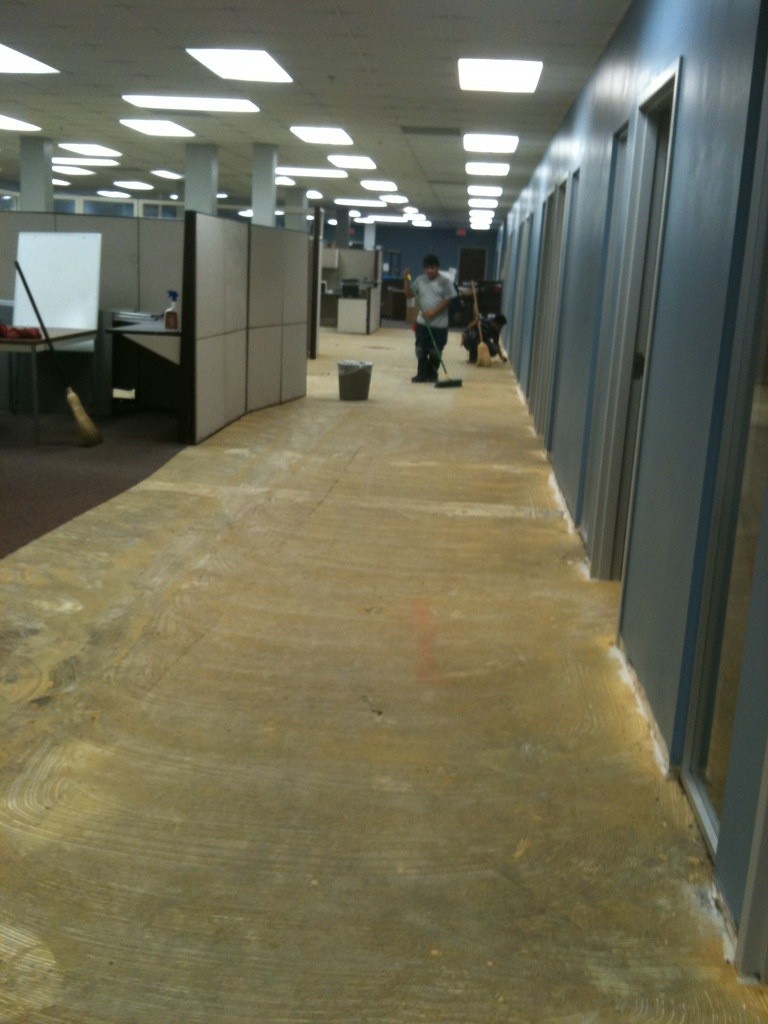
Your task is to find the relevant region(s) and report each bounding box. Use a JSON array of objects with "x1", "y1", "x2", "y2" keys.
[
  {"x1": 470, "y1": 354, "x2": 477, "y2": 363},
  {"x1": 411, "y1": 371, "x2": 430, "y2": 383},
  {"x1": 428, "y1": 371, "x2": 437, "y2": 381}
]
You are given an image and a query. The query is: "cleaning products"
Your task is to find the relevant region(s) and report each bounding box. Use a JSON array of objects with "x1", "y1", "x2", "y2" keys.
[{"x1": 162, "y1": 287, "x2": 178, "y2": 330}]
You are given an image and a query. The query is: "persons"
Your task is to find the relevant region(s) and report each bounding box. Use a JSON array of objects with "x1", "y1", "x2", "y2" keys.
[
  {"x1": 462, "y1": 313, "x2": 508, "y2": 363},
  {"x1": 403, "y1": 255, "x2": 451, "y2": 382}
]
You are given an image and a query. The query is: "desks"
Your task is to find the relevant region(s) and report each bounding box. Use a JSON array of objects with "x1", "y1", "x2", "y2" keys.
[
  {"x1": 0, "y1": 326, "x2": 98, "y2": 446},
  {"x1": 107, "y1": 316, "x2": 181, "y2": 378}
]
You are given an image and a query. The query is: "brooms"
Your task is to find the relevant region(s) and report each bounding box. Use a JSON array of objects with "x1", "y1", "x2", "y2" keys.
[
  {"x1": 11, "y1": 257, "x2": 101, "y2": 448},
  {"x1": 409, "y1": 273, "x2": 463, "y2": 388},
  {"x1": 471, "y1": 287, "x2": 491, "y2": 369}
]
